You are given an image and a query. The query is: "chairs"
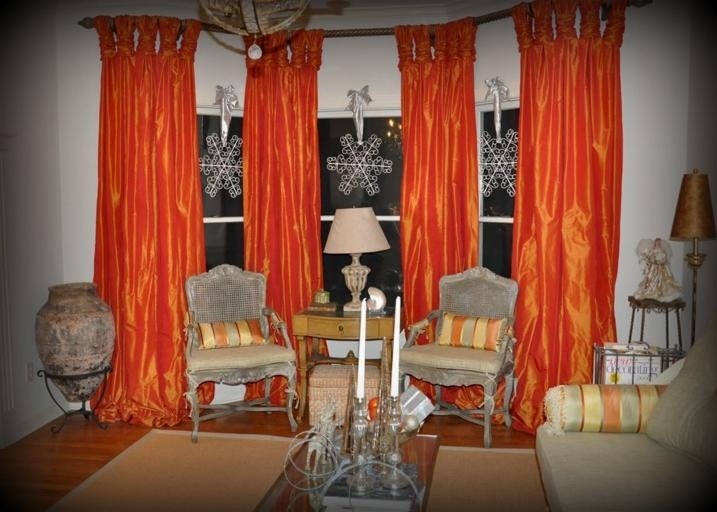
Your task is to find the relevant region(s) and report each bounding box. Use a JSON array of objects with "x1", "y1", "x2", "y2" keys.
[
  {"x1": 183, "y1": 263, "x2": 298, "y2": 444},
  {"x1": 398, "y1": 267, "x2": 519, "y2": 448},
  {"x1": 536, "y1": 339, "x2": 717, "y2": 511}
]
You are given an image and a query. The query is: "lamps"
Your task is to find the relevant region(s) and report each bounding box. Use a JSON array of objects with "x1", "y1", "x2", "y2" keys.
[
  {"x1": 670, "y1": 173, "x2": 717, "y2": 347},
  {"x1": 322, "y1": 207, "x2": 391, "y2": 311},
  {"x1": 198, "y1": 0, "x2": 314, "y2": 61}
]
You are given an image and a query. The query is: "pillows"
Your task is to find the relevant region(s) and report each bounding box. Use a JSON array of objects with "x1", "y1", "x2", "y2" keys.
[
  {"x1": 196, "y1": 320, "x2": 268, "y2": 351},
  {"x1": 543, "y1": 385, "x2": 669, "y2": 434},
  {"x1": 436, "y1": 311, "x2": 507, "y2": 353}
]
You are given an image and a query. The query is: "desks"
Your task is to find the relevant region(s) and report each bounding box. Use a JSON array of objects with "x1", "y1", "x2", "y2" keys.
[{"x1": 292, "y1": 301, "x2": 395, "y2": 433}]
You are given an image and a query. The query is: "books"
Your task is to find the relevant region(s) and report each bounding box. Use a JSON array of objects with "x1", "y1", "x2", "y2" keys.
[
  {"x1": 397, "y1": 382, "x2": 437, "y2": 424},
  {"x1": 602, "y1": 337, "x2": 663, "y2": 386}
]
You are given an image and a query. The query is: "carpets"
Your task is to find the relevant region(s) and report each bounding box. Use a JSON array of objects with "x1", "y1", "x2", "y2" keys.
[{"x1": 50, "y1": 427, "x2": 550, "y2": 511}]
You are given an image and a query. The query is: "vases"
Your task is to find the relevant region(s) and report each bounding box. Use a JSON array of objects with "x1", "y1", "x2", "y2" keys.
[{"x1": 35, "y1": 282, "x2": 117, "y2": 403}]
